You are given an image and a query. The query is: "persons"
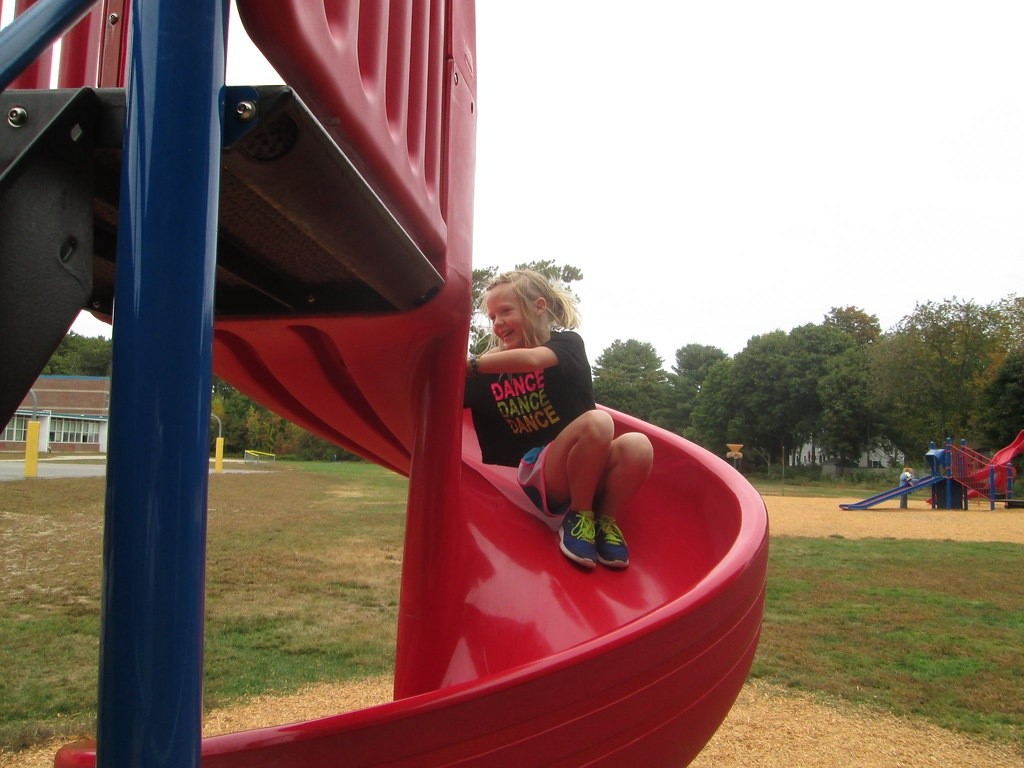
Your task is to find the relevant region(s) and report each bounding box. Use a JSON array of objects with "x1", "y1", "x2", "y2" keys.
[
  {"x1": 900, "y1": 468, "x2": 919, "y2": 509},
  {"x1": 464, "y1": 269, "x2": 655, "y2": 571}
]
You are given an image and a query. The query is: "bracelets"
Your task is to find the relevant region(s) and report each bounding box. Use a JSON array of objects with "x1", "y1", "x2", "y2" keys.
[{"x1": 468, "y1": 355, "x2": 480, "y2": 369}]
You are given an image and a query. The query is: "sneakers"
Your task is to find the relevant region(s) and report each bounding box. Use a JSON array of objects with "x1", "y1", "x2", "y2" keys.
[{"x1": 559, "y1": 510, "x2": 629, "y2": 569}]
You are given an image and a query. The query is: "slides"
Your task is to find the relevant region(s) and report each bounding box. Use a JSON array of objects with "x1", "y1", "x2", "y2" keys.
[
  {"x1": 55, "y1": 405, "x2": 769, "y2": 768},
  {"x1": 838, "y1": 474, "x2": 945, "y2": 510},
  {"x1": 927, "y1": 430, "x2": 1024, "y2": 504}
]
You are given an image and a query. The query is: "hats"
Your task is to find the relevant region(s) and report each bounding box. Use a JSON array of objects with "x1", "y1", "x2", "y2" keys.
[{"x1": 905, "y1": 468, "x2": 912, "y2": 472}]
